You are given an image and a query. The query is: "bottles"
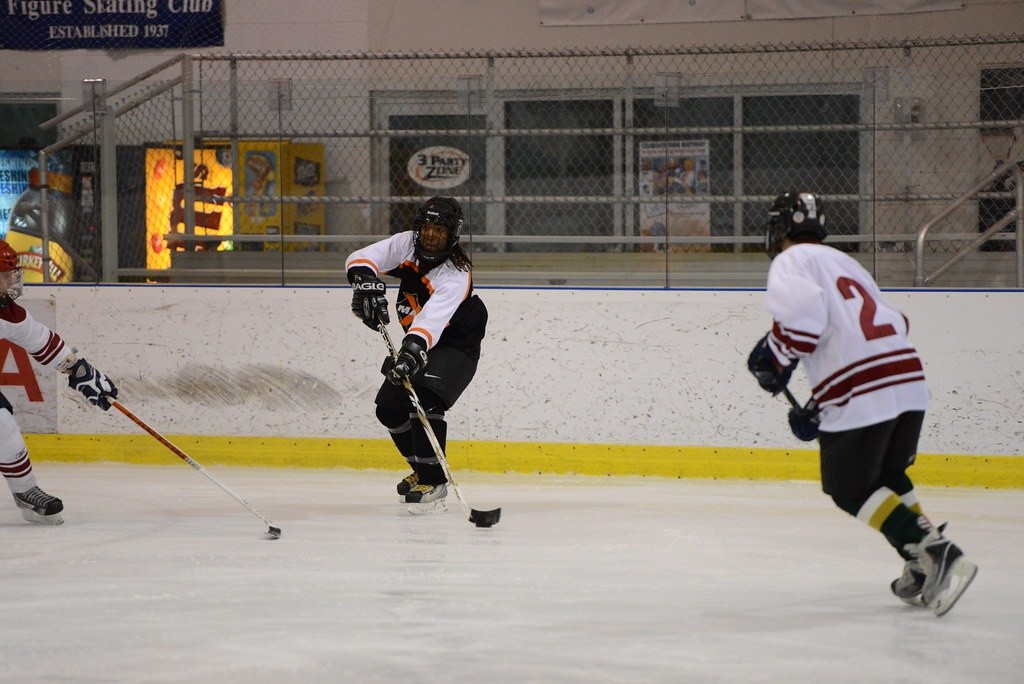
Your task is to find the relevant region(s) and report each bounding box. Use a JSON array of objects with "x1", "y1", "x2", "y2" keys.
[{"x1": 5, "y1": 168, "x2": 75, "y2": 283}]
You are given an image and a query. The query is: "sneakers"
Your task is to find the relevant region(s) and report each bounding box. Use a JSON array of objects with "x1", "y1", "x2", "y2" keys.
[
  {"x1": 890, "y1": 558, "x2": 928, "y2": 607},
  {"x1": 12, "y1": 484, "x2": 65, "y2": 526},
  {"x1": 903, "y1": 527, "x2": 978, "y2": 616},
  {"x1": 397, "y1": 469, "x2": 424, "y2": 496},
  {"x1": 404, "y1": 482, "x2": 450, "y2": 515}
]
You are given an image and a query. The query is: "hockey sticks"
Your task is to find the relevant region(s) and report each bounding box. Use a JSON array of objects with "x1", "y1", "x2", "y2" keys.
[
  {"x1": 104, "y1": 395, "x2": 282, "y2": 539},
  {"x1": 374, "y1": 316, "x2": 502, "y2": 526},
  {"x1": 782, "y1": 386, "x2": 947, "y2": 535}
]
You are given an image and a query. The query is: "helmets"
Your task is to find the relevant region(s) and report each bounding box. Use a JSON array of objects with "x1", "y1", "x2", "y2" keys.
[
  {"x1": 413, "y1": 196, "x2": 464, "y2": 261},
  {"x1": 1, "y1": 241, "x2": 24, "y2": 300},
  {"x1": 764, "y1": 190, "x2": 828, "y2": 262}
]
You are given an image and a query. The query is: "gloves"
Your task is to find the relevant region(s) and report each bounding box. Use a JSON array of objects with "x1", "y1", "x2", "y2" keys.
[
  {"x1": 787, "y1": 403, "x2": 821, "y2": 442},
  {"x1": 67, "y1": 357, "x2": 120, "y2": 411},
  {"x1": 381, "y1": 340, "x2": 428, "y2": 386},
  {"x1": 748, "y1": 339, "x2": 800, "y2": 398},
  {"x1": 347, "y1": 266, "x2": 391, "y2": 332}
]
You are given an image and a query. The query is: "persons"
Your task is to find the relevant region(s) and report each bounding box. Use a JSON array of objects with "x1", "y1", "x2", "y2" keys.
[
  {"x1": 346, "y1": 197, "x2": 488, "y2": 514},
  {"x1": 0, "y1": 241, "x2": 118, "y2": 524},
  {"x1": 748, "y1": 192, "x2": 979, "y2": 615}
]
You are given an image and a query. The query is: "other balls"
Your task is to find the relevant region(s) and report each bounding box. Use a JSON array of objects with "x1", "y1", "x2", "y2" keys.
[{"x1": 476, "y1": 514, "x2": 493, "y2": 527}]
